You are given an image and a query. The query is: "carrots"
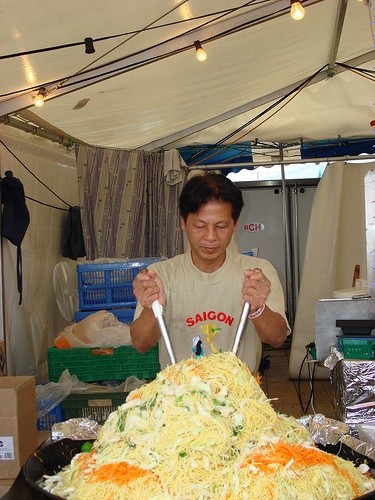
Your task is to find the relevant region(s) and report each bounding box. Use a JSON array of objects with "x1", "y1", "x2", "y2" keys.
[
  {"x1": 236, "y1": 438, "x2": 360, "y2": 497},
  {"x1": 75, "y1": 448, "x2": 155, "y2": 488}
]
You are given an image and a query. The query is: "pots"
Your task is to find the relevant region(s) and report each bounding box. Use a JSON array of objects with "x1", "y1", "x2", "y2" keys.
[{"x1": 22, "y1": 434, "x2": 375, "y2": 500}]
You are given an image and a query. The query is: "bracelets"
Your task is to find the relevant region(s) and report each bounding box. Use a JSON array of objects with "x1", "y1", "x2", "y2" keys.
[{"x1": 247, "y1": 302, "x2": 266, "y2": 319}]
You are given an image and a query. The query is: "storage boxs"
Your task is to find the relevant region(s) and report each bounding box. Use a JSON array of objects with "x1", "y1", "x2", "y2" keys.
[
  {"x1": 61, "y1": 390, "x2": 127, "y2": 424},
  {"x1": 46, "y1": 346, "x2": 160, "y2": 383},
  {"x1": 77, "y1": 257, "x2": 164, "y2": 310},
  {"x1": 75, "y1": 310, "x2": 134, "y2": 325},
  {"x1": 0, "y1": 376, "x2": 36, "y2": 480},
  {"x1": 37, "y1": 398, "x2": 60, "y2": 431}
]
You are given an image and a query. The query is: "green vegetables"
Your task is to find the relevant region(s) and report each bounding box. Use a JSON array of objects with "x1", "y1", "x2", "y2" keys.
[{"x1": 80, "y1": 377, "x2": 243, "y2": 458}]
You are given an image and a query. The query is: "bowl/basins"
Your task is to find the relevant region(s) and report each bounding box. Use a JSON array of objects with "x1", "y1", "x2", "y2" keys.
[{"x1": 335, "y1": 320, "x2": 375, "y2": 335}]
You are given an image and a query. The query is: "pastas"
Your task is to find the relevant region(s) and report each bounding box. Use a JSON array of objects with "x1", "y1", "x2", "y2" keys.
[{"x1": 45, "y1": 352, "x2": 375, "y2": 500}]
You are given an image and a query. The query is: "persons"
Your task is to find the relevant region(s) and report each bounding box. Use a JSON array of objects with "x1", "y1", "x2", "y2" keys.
[{"x1": 129, "y1": 170, "x2": 292, "y2": 378}]
